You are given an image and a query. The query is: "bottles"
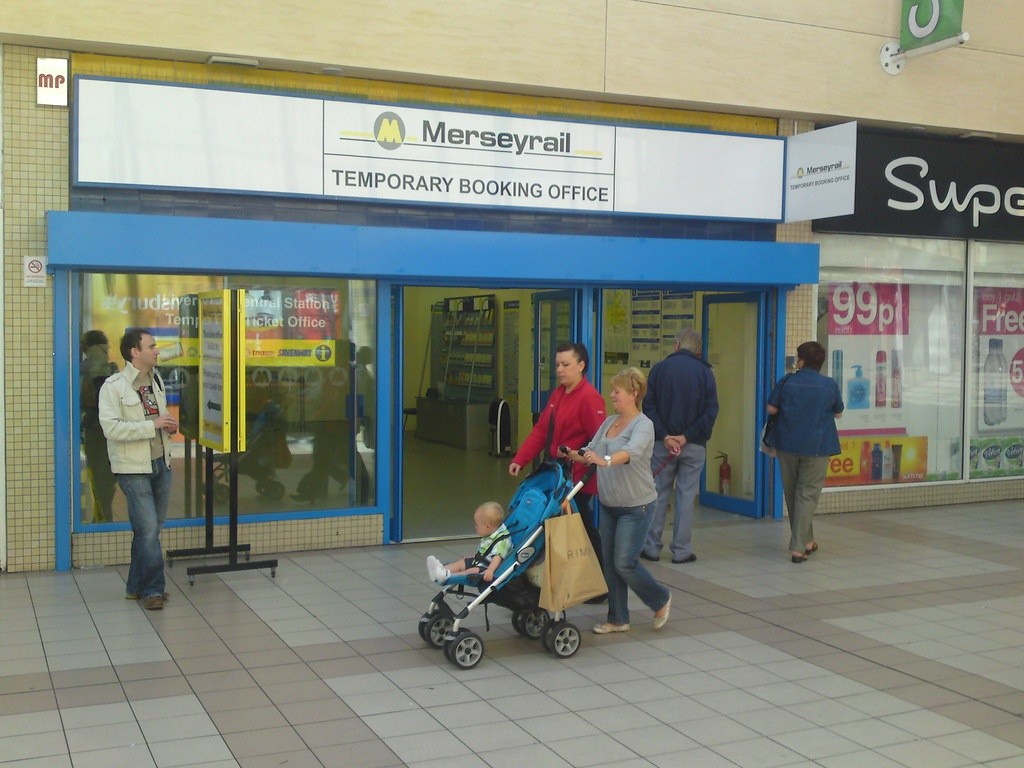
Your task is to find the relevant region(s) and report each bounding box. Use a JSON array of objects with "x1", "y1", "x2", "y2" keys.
[
  {"x1": 871, "y1": 443, "x2": 883, "y2": 480},
  {"x1": 860, "y1": 441, "x2": 871, "y2": 482},
  {"x1": 984, "y1": 338, "x2": 1008, "y2": 426}
]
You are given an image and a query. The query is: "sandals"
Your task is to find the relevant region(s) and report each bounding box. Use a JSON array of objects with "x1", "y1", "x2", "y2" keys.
[
  {"x1": 806, "y1": 542, "x2": 818, "y2": 555},
  {"x1": 792, "y1": 553, "x2": 807, "y2": 563}
]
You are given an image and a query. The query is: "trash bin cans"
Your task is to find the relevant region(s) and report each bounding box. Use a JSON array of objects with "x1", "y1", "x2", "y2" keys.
[{"x1": 488, "y1": 397, "x2": 512, "y2": 459}]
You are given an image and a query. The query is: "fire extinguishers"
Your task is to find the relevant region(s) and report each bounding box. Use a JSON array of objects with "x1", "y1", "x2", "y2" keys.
[{"x1": 714, "y1": 450, "x2": 731, "y2": 497}]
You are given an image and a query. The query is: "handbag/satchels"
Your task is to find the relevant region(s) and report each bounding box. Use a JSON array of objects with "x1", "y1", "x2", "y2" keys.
[
  {"x1": 759, "y1": 420, "x2": 777, "y2": 458},
  {"x1": 537, "y1": 497, "x2": 609, "y2": 612}
]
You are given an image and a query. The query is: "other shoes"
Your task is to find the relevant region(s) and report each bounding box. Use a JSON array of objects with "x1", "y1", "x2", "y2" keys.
[
  {"x1": 593, "y1": 623, "x2": 630, "y2": 634},
  {"x1": 640, "y1": 550, "x2": 659, "y2": 561},
  {"x1": 427, "y1": 556, "x2": 437, "y2": 582},
  {"x1": 145, "y1": 596, "x2": 163, "y2": 609},
  {"x1": 653, "y1": 593, "x2": 671, "y2": 630},
  {"x1": 125, "y1": 591, "x2": 170, "y2": 601},
  {"x1": 672, "y1": 554, "x2": 697, "y2": 564},
  {"x1": 435, "y1": 559, "x2": 447, "y2": 584}
]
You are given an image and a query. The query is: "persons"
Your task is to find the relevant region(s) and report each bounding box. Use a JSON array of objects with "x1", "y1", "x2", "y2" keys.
[
  {"x1": 767, "y1": 341, "x2": 844, "y2": 563},
  {"x1": 98, "y1": 329, "x2": 178, "y2": 610},
  {"x1": 80, "y1": 330, "x2": 377, "y2": 524},
  {"x1": 508, "y1": 327, "x2": 720, "y2": 634},
  {"x1": 427, "y1": 502, "x2": 514, "y2": 586}
]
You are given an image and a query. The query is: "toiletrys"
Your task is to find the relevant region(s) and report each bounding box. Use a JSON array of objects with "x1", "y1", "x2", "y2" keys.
[
  {"x1": 860, "y1": 441, "x2": 872, "y2": 483},
  {"x1": 871, "y1": 443, "x2": 883, "y2": 481},
  {"x1": 881, "y1": 440, "x2": 894, "y2": 480},
  {"x1": 891, "y1": 445, "x2": 903, "y2": 480},
  {"x1": 846, "y1": 365, "x2": 871, "y2": 409}
]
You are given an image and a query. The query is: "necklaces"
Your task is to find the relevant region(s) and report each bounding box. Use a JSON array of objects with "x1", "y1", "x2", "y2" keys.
[{"x1": 615, "y1": 412, "x2": 636, "y2": 426}]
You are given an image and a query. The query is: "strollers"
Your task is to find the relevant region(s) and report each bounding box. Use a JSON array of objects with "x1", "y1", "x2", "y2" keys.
[
  {"x1": 201, "y1": 391, "x2": 293, "y2": 505},
  {"x1": 418, "y1": 446, "x2": 597, "y2": 670}
]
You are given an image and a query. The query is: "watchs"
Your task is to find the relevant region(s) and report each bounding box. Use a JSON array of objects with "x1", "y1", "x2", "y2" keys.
[{"x1": 603, "y1": 455, "x2": 612, "y2": 468}]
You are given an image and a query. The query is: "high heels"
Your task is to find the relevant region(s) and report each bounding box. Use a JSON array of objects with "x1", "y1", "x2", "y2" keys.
[{"x1": 290, "y1": 493, "x2": 316, "y2": 505}]
